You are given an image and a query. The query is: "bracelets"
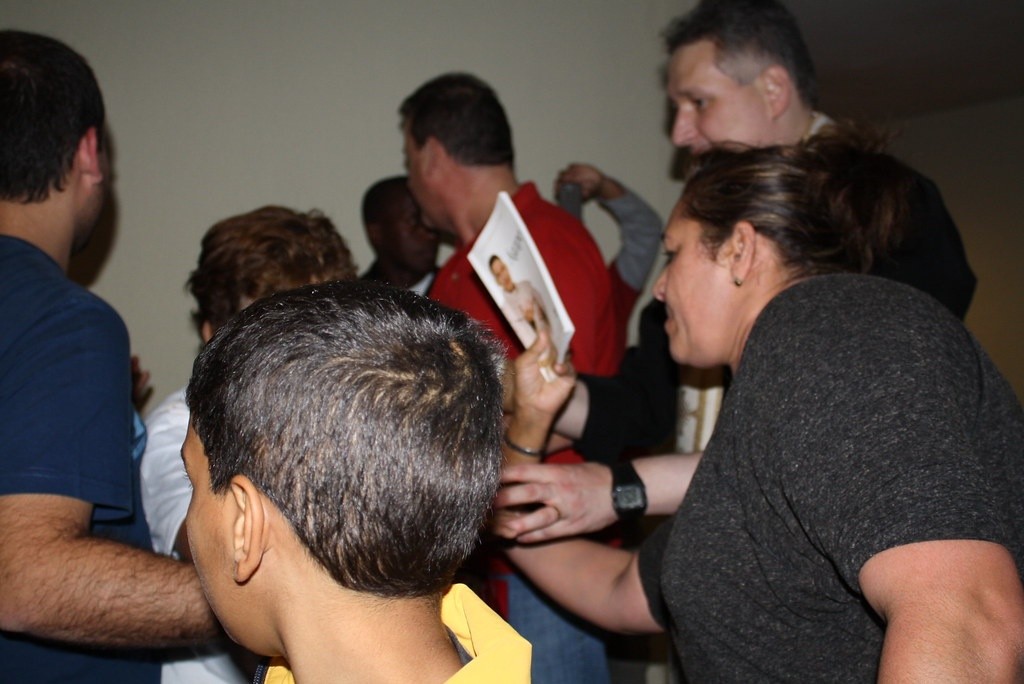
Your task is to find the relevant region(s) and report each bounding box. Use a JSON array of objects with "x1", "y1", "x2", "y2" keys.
[{"x1": 503, "y1": 433, "x2": 543, "y2": 457}]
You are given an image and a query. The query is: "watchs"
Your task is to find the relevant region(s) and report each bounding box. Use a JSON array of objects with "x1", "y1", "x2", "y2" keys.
[{"x1": 609, "y1": 462, "x2": 648, "y2": 526}]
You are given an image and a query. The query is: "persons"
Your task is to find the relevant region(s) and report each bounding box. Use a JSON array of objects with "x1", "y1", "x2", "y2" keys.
[
  {"x1": 177, "y1": 283, "x2": 536, "y2": 684},
  {"x1": 554, "y1": 158, "x2": 662, "y2": 355},
  {"x1": 489, "y1": 256, "x2": 552, "y2": 336},
  {"x1": 494, "y1": 121, "x2": 1022, "y2": 684},
  {"x1": 141, "y1": 202, "x2": 365, "y2": 683},
  {"x1": 357, "y1": 167, "x2": 447, "y2": 307},
  {"x1": 397, "y1": 72, "x2": 620, "y2": 684},
  {"x1": 0, "y1": 24, "x2": 220, "y2": 684},
  {"x1": 494, "y1": 1, "x2": 979, "y2": 542}
]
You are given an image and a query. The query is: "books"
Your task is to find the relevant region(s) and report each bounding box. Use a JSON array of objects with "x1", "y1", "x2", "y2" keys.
[{"x1": 466, "y1": 191, "x2": 575, "y2": 383}]
